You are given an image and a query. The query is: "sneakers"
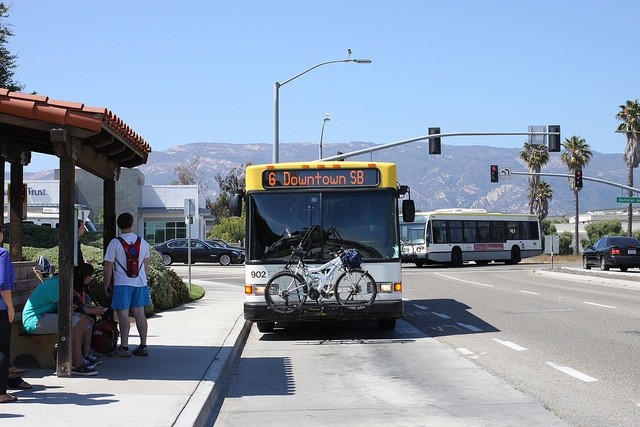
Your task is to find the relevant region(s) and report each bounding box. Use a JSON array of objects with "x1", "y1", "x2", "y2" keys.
[
  {"x1": 16, "y1": 379, "x2": 30, "y2": 388},
  {"x1": 0, "y1": 394, "x2": 16, "y2": 403},
  {"x1": 8, "y1": 368, "x2": 25, "y2": 379},
  {"x1": 112, "y1": 347, "x2": 131, "y2": 357},
  {"x1": 72, "y1": 353, "x2": 103, "y2": 369},
  {"x1": 133, "y1": 347, "x2": 148, "y2": 356},
  {"x1": 71, "y1": 365, "x2": 98, "y2": 376}
]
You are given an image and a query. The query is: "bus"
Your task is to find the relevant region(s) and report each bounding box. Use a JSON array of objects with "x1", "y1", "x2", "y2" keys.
[
  {"x1": 231, "y1": 159, "x2": 414, "y2": 331},
  {"x1": 399, "y1": 207, "x2": 542, "y2": 266}
]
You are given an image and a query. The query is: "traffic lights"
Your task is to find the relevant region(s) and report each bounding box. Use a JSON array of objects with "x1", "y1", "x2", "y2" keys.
[
  {"x1": 428, "y1": 126, "x2": 441, "y2": 153},
  {"x1": 575, "y1": 169, "x2": 583, "y2": 187},
  {"x1": 491, "y1": 164, "x2": 498, "y2": 182},
  {"x1": 548, "y1": 125, "x2": 561, "y2": 152}
]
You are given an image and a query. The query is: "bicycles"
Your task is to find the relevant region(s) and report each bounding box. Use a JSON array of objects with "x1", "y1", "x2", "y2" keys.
[{"x1": 264, "y1": 224, "x2": 377, "y2": 315}]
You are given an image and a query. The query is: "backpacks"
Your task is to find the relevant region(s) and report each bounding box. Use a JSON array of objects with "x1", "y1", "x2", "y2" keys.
[
  {"x1": 92, "y1": 308, "x2": 119, "y2": 353},
  {"x1": 115, "y1": 235, "x2": 146, "y2": 278}
]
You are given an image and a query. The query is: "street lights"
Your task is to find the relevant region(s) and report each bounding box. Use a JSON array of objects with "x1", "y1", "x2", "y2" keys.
[
  {"x1": 318, "y1": 112, "x2": 331, "y2": 158},
  {"x1": 273, "y1": 48, "x2": 372, "y2": 163}
]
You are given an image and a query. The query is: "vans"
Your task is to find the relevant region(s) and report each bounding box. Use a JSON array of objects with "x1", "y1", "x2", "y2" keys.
[{"x1": 4, "y1": 207, "x2": 96, "y2": 231}]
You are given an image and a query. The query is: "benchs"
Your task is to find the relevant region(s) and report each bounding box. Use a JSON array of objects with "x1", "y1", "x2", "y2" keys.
[{"x1": 9, "y1": 262, "x2": 57, "y2": 368}]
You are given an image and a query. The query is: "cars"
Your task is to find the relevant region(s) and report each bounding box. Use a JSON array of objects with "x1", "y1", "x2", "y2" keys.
[
  {"x1": 582, "y1": 235, "x2": 640, "y2": 269},
  {"x1": 213, "y1": 238, "x2": 246, "y2": 264},
  {"x1": 151, "y1": 237, "x2": 240, "y2": 264}
]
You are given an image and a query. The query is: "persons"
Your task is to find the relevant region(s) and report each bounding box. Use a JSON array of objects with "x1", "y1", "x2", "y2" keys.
[
  {"x1": 0, "y1": 224, "x2": 17, "y2": 403},
  {"x1": 73, "y1": 263, "x2": 106, "y2": 334},
  {"x1": 21, "y1": 269, "x2": 99, "y2": 376},
  {"x1": 103, "y1": 212, "x2": 150, "y2": 357},
  {"x1": 78, "y1": 220, "x2": 85, "y2": 265}
]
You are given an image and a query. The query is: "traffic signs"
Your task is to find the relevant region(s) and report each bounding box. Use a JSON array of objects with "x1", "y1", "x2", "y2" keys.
[{"x1": 617, "y1": 196, "x2": 640, "y2": 202}]
